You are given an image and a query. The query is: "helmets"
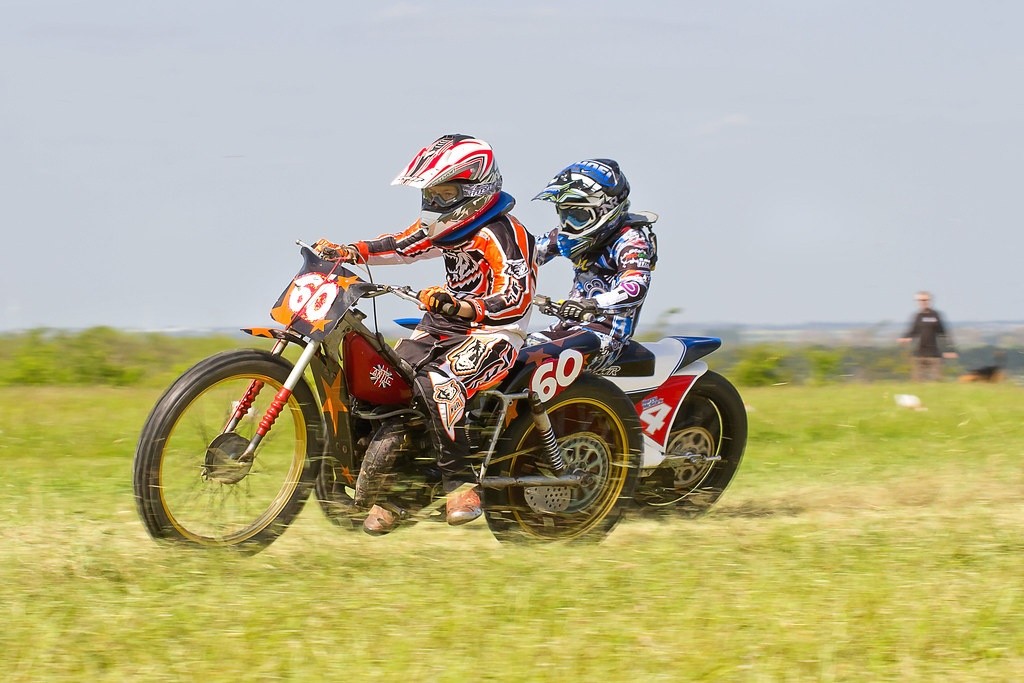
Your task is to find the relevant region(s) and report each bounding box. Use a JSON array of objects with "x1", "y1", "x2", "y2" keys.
[
  {"x1": 531, "y1": 159, "x2": 630, "y2": 259},
  {"x1": 390, "y1": 134, "x2": 504, "y2": 240}
]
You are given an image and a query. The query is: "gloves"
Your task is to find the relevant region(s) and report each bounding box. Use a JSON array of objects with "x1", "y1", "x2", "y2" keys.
[
  {"x1": 311, "y1": 239, "x2": 349, "y2": 263},
  {"x1": 416, "y1": 286, "x2": 461, "y2": 316},
  {"x1": 559, "y1": 298, "x2": 600, "y2": 322}
]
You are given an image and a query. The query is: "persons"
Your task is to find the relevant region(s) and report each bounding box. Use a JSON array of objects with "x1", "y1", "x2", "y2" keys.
[
  {"x1": 898, "y1": 292, "x2": 958, "y2": 384},
  {"x1": 312, "y1": 134, "x2": 538, "y2": 538},
  {"x1": 523, "y1": 158, "x2": 658, "y2": 377}
]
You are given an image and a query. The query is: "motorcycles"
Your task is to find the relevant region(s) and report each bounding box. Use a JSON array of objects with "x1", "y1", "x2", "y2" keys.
[
  {"x1": 133, "y1": 238, "x2": 644, "y2": 556},
  {"x1": 315, "y1": 295, "x2": 748, "y2": 529}
]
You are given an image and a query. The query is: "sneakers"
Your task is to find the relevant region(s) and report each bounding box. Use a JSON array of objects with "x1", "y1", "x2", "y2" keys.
[
  {"x1": 363, "y1": 507, "x2": 400, "y2": 536},
  {"x1": 446, "y1": 489, "x2": 483, "y2": 526}
]
